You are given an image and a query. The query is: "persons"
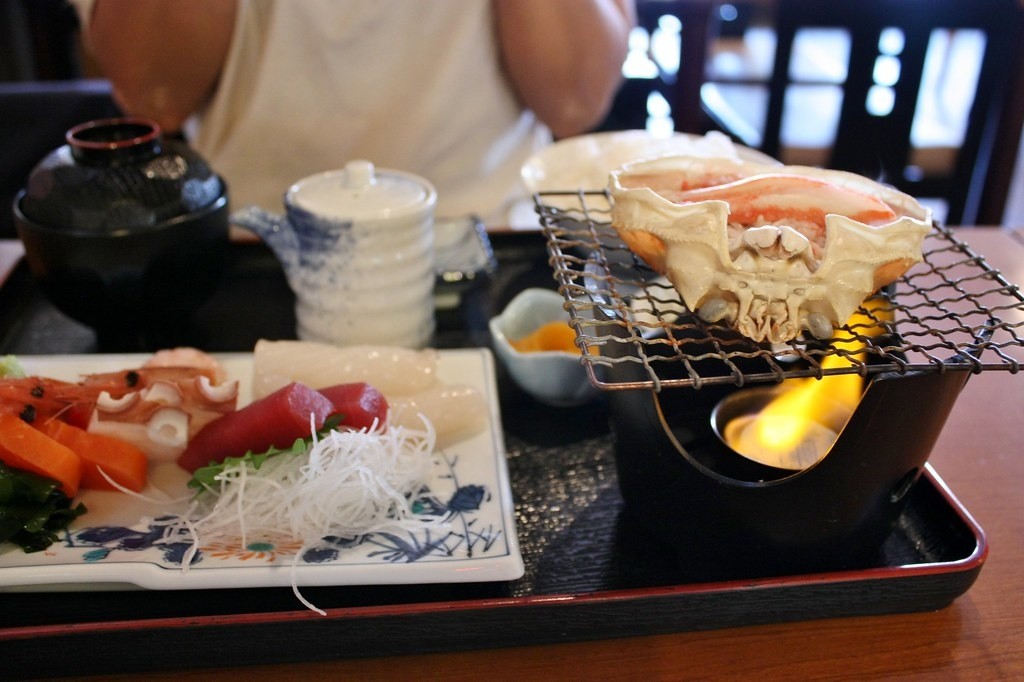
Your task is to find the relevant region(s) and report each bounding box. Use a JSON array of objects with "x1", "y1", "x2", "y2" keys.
[{"x1": 79, "y1": 0, "x2": 636, "y2": 233}]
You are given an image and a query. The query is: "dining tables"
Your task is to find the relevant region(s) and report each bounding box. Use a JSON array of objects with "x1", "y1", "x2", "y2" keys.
[{"x1": 2, "y1": 220, "x2": 1024, "y2": 682}]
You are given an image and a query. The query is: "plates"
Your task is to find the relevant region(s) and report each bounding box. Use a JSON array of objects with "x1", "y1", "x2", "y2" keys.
[
  {"x1": 1, "y1": 348, "x2": 523, "y2": 592},
  {"x1": 228, "y1": 203, "x2": 496, "y2": 282}
]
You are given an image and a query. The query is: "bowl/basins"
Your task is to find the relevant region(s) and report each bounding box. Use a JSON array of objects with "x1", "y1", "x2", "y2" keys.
[
  {"x1": 490, "y1": 288, "x2": 611, "y2": 410},
  {"x1": 519, "y1": 127, "x2": 788, "y2": 222},
  {"x1": 19, "y1": 116, "x2": 225, "y2": 239},
  {"x1": 8, "y1": 188, "x2": 230, "y2": 340}
]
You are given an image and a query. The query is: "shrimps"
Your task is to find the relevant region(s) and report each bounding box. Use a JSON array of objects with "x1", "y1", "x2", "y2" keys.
[{"x1": 0, "y1": 366, "x2": 224, "y2": 427}]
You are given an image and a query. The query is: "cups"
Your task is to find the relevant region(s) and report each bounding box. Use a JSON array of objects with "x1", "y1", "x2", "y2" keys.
[{"x1": 283, "y1": 159, "x2": 436, "y2": 349}]
